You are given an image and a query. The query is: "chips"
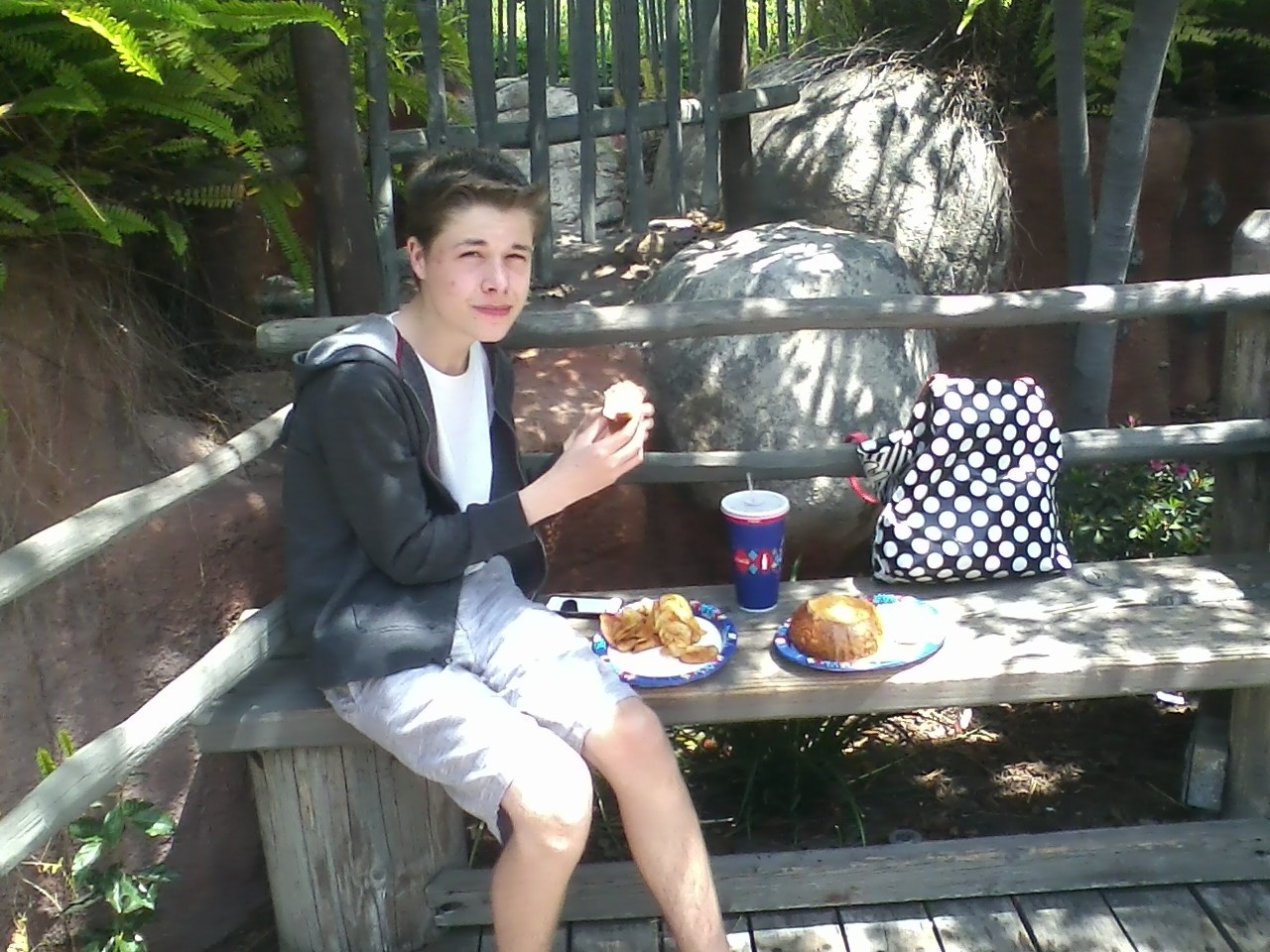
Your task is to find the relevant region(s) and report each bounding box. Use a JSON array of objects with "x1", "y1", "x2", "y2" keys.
[{"x1": 600, "y1": 593, "x2": 719, "y2": 664}]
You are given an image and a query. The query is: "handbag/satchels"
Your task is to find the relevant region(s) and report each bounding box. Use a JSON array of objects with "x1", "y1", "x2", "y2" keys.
[{"x1": 845, "y1": 372, "x2": 1073, "y2": 584}]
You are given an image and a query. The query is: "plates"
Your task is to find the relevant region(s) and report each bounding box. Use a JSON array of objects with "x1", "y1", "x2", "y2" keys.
[
  {"x1": 776, "y1": 593, "x2": 946, "y2": 673},
  {"x1": 591, "y1": 598, "x2": 738, "y2": 688}
]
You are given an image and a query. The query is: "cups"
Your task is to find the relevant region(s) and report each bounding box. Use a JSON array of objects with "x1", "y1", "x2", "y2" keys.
[{"x1": 721, "y1": 490, "x2": 790, "y2": 614}]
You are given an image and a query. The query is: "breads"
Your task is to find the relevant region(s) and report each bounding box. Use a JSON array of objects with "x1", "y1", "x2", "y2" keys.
[
  {"x1": 787, "y1": 595, "x2": 882, "y2": 661},
  {"x1": 602, "y1": 380, "x2": 643, "y2": 421}
]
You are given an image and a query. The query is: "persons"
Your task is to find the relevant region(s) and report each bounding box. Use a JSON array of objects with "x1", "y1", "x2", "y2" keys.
[{"x1": 274, "y1": 144, "x2": 743, "y2": 951}]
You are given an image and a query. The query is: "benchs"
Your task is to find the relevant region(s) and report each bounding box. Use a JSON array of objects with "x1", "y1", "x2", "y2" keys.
[{"x1": 192, "y1": 553, "x2": 1269, "y2": 951}]
